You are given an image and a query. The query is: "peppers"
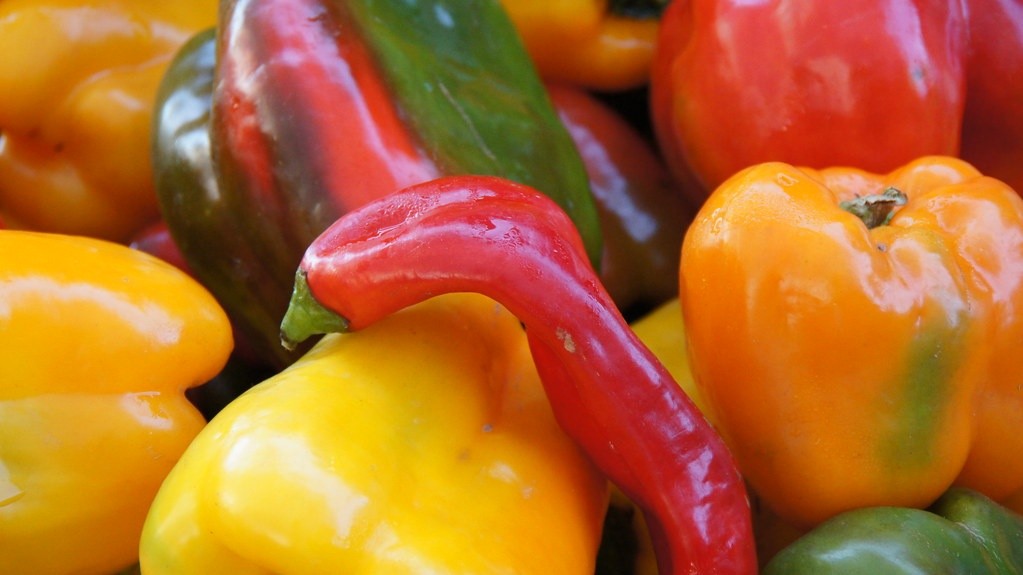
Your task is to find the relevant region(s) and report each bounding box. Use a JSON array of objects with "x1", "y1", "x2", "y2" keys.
[{"x1": 0, "y1": 0, "x2": 1023, "y2": 575}]
